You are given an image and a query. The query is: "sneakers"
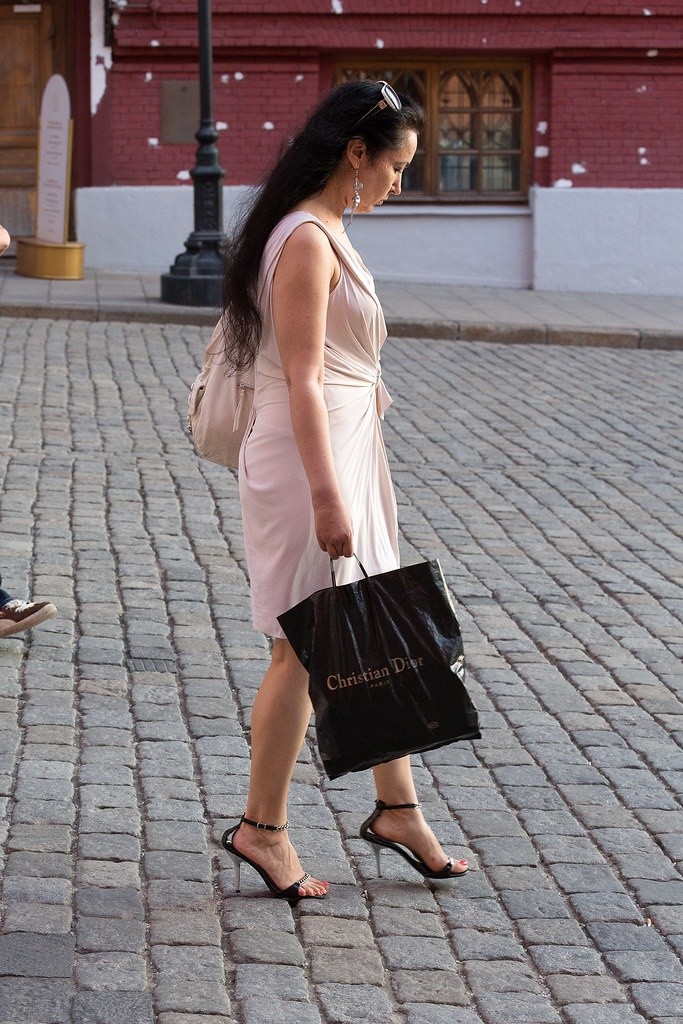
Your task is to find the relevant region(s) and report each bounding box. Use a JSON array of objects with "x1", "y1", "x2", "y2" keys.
[{"x1": 0, "y1": 599, "x2": 57, "y2": 637}]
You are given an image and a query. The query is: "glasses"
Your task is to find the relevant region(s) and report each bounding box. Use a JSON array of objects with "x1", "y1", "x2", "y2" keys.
[{"x1": 352, "y1": 81, "x2": 402, "y2": 130}]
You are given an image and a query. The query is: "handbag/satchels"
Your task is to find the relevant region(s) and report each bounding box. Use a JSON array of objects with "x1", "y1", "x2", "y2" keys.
[
  {"x1": 186, "y1": 264, "x2": 256, "y2": 470},
  {"x1": 276, "y1": 552, "x2": 482, "y2": 783}
]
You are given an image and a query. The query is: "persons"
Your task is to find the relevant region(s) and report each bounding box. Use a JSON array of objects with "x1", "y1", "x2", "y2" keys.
[
  {"x1": 0, "y1": 575, "x2": 57, "y2": 636},
  {"x1": 222, "y1": 79, "x2": 471, "y2": 896}
]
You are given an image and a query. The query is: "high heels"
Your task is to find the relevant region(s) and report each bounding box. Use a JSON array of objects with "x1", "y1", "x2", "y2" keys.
[
  {"x1": 221, "y1": 813, "x2": 328, "y2": 900},
  {"x1": 359, "y1": 798, "x2": 469, "y2": 880}
]
